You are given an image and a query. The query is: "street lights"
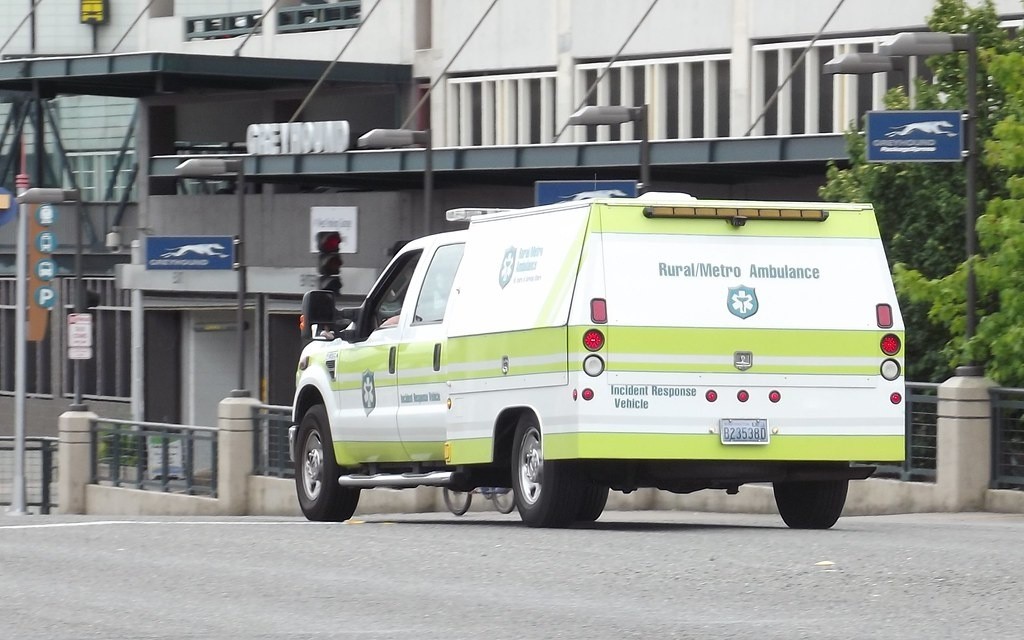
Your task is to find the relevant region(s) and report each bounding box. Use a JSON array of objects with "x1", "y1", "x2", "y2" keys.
[
  {"x1": 821, "y1": 52, "x2": 913, "y2": 99},
  {"x1": 12, "y1": 187, "x2": 85, "y2": 406},
  {"x1": 172, "y1": 158, "x2": 248, "y2": 388},
  {"x1": 877, "y1": 31, "x2": 982, "y2": 351},
  {"x1": 569, "y1": 104, "x2": 652, "y2": 190},
  {"x1": 355, "y1": 128, "x2": 436, "y2": 236}
]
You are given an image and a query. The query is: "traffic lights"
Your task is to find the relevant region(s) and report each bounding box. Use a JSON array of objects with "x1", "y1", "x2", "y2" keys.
[{"x1": 315, "y1": 230, "x2": 344, "y2": 295}]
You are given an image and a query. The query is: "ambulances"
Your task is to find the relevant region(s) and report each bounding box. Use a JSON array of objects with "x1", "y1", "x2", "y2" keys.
[{"x1": 284, "y1": 191, "x2": 908, "y2": 529}]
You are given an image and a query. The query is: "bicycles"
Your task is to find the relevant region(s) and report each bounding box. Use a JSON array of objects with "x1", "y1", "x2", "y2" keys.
[{"x1": 440, "y1": 482, "x2": 515, "y2": 516}]
